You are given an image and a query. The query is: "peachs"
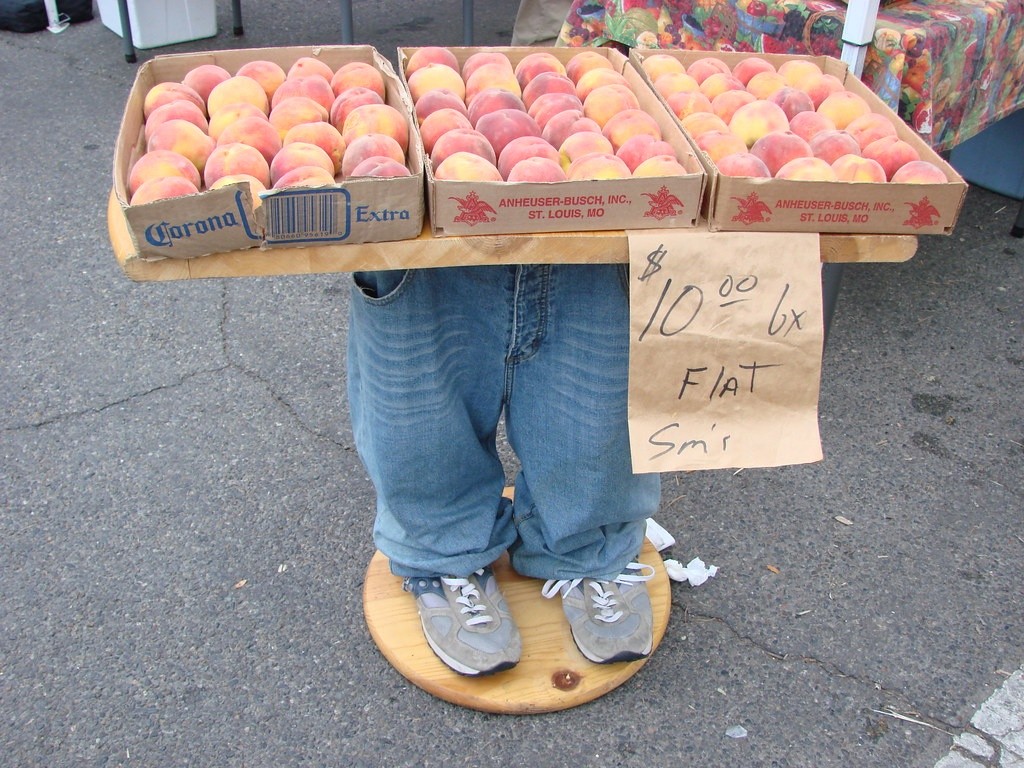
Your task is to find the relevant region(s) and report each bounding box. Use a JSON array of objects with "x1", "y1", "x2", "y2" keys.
[
  {"x1": 129, "y1": 55, "x2": 412, "y2": 213},
  {"x1": 407, "y1": 46, "x2": 686, "y2": 183},
  {"x1": 644, "y1": 54, "x2": 948, "y2": 185}
]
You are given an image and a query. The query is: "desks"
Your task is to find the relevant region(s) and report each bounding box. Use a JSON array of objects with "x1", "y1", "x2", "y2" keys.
[
  {"x1": 106, "y1": 187, "x2": 922, "y2": 713},
  {"x1": 553, "y1": 0, "x2": 1024, "y2": 355}
]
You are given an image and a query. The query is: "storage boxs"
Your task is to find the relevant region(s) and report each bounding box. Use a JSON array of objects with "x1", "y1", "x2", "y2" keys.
[{"x1": 112, "y1": 41, "x2": 969, "y2": 265}]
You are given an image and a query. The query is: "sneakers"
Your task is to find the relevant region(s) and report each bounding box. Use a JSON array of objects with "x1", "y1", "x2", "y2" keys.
[
  {"x1": 405, "y1": 564, "x2": 520, "y2": 677},
  {"x1": 542, "y1": 554, "x2": 653, "y2": 664}
]
